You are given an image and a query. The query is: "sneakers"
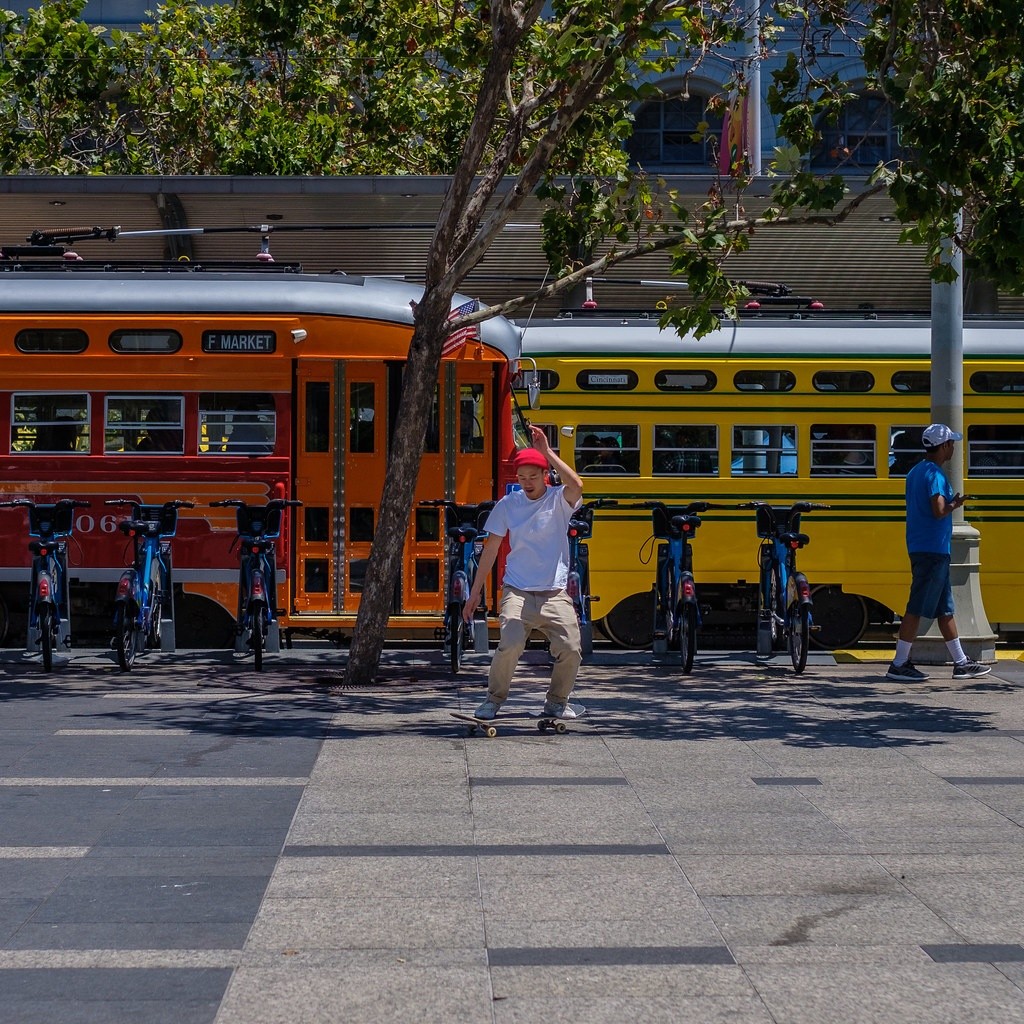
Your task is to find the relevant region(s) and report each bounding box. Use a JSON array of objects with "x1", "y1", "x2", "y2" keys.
[
  {"x1": 952, "y1": 656, "x2": 992, "y2": 679},
  {"x1": 886, "y1": 660, "x2": 929, "y2": 681}
]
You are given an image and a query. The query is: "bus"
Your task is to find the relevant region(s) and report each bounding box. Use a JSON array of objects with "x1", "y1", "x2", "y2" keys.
[{"x1": 0, "y1": 220, "x2": 1024, "y2": 649}]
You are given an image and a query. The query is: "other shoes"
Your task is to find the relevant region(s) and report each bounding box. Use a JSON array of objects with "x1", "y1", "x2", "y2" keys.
[
  {"x1": 544, "y1": 701, "x2": 577, "y2": 720},
  {"x1": 475, "y1": 700, "x2": 501, "y2": 721}
]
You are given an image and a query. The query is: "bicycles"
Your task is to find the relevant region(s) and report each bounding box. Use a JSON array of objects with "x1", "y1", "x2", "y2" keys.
[
  {"x1": 566, "y1": 496, "x2": 620, "y2": 627},
  {"x1": 735, "y1": 498, "x2": 833, "y2": 676},
  {"x1": 0, "y1": 497, "x2": 94, "y2": 673},
  {"x1": 416, "y1": 498, "x2": 502, "y2": 675},
  {"x1": 103, "y1": 496, "x2": 196, "y2": 674},
  {"x1": 206, "y1": 497, "x2": 305, "y2": 672},
  {"x1": 632, "y1": 500, "x2": 728, "y2": 676}
]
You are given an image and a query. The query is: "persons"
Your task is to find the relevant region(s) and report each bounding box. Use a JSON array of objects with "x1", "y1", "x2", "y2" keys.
[
  {"x1": 885, "y1": 424, "x2": 992, "y2": 680},
  {"x1": 462, "y1": 424, "x2": 585, "y2": 720},
  {"x1": 10, "y1": 426, "x2": 19, "y2": 451},
  {"x1": 32, "y1": 416, "x2": 77, "y2": 451},
  {"x1": 575, "y1": 422, "x2": 926, "y2": 476},
  {"x1": 225, "y1": 404, "x2": 272, "y2": 452},
  {"x1": 136, "y1": 407, "x2": 177, "y2": 453}
]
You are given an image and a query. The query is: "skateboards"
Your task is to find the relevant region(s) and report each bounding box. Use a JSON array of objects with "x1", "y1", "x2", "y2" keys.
[{"x1": 449, "y1": 702, "x2": 587, "y2": 739}]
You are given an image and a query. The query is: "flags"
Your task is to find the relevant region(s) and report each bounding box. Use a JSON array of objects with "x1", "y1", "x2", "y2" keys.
[{"x1": 441, "y1": 297, "x2": 480, "y2": 358}]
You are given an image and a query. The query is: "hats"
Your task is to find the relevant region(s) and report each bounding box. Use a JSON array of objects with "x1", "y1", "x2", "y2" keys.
[
  {"x1": 514, "y1": 448, "x2": 550, "y2": 472},
  {"x1": 921, "y1": 423, "x2": 962, "y2": 446}
]
